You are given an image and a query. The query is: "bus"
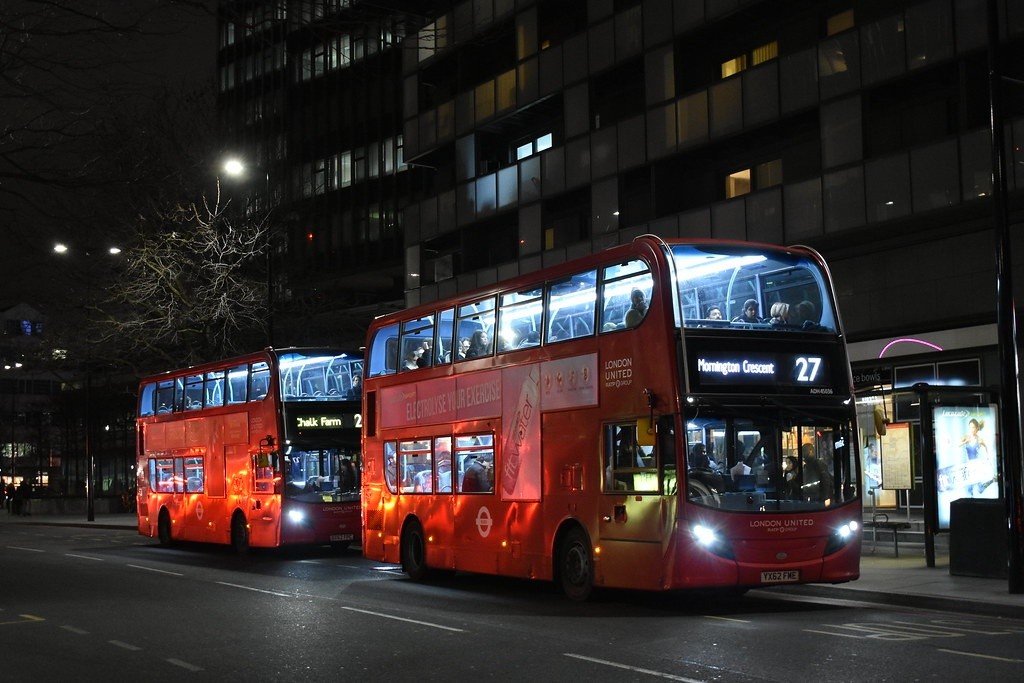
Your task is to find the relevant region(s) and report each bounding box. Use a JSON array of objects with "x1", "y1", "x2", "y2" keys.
[
  {"x1": 134, "y1": 346, "x2": 371, "y2": 559},
  {"x1": 356, "y1": 231, "x2": 890, "y2": 609}
]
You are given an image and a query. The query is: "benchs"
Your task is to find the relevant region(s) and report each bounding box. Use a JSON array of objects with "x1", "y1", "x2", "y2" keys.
[{"x1": 862, "y1": 521, "x2": 912, "y2": 558}]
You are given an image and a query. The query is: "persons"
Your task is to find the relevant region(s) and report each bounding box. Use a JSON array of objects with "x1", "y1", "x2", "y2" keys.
[
  {"x1": 348, "y1": 375, "x2": 362, "y2": 402},
  {"x1": 615, "y1": 427, "x2": 647, "y2": 491},
  {"x1": 783, "y1": 456, "x2": 800, "y2": 501},
  {"x1": 402, "y1": 338, "x2": 443, "y2": 371},
  {"x1": 957, "y1": 419, "x2": 998, "y2": 499},
  {"x1": 624, "y1": 288, "x2": 647, "y2": 328},
  {"x1": 339, "y1": 459, "x2": 356, "y2": 493},
  {"x1": 688, "y1": 443, "x2": 725, "y2": 493},
  {"x1": 387, "y1": 452, "x2": 412, "y2": 487},
  {"x1": 6, "y1": 481, "x2": 25, "y2": 516},
  {"x1": 445, "y1": 329, "x2": 489, "y2": 363},
  {"x1": 802, "y1": 443, "x2": 833, "y2": 507},
  {"x1": 463, "y1": 455, "x2": 493, "y2": 492},
  {"x1": 426, "y1": 451, "x2": 452, "y2": 493},
  {"x1": 868, "y1": 443, "x2": 878, "y2": 463},
  {"x1": 521, "y1": 331, "x2": 541, "y2": 347},
  {"x1": 46, "y1": 459, "x2": 68, "y2": 516},
  {"x1": 698, "y1": 299, "x2": 819, "y2": 329},
  {"x1": 179, "y1": 396, "x2": 192, "y2": 412}
]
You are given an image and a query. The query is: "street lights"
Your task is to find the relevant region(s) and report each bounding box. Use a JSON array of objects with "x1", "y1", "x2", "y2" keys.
[
  {"x1": 218, "y1": 152, "x2": 275, "y2": 348},
  {"x1": 52, "y1": 241, "x2": 124, "y2": 522}
]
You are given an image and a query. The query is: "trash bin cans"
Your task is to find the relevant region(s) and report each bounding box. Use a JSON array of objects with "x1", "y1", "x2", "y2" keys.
[{"x1": 950, "y1": 497, "x2": 1008, "y2": 580}]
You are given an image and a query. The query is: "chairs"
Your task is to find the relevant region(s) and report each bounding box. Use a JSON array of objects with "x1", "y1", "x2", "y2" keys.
[
  {"x1": 283, "y1": 389, "x2": 343, "y2": 401},
  {"x1": 414, "y1": 470, "x2": 465, "y2": 493},
  {"x1": 188, "y1": 477, "x2": 203, "y2": 492},
  {"x1": 152, "y1": 400, "x2": 223, "y2": 415}
]
